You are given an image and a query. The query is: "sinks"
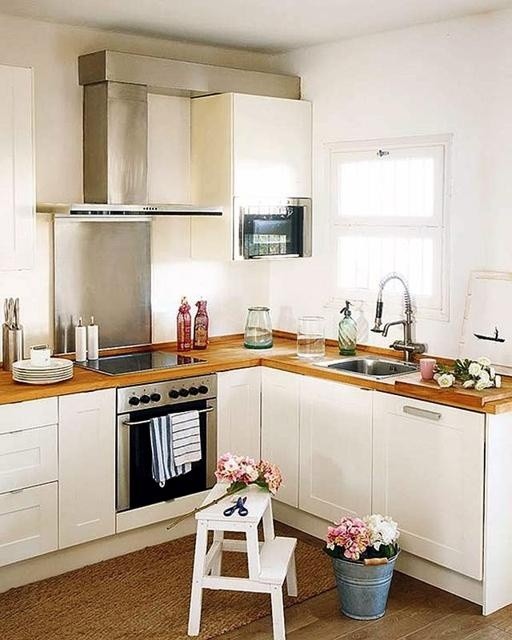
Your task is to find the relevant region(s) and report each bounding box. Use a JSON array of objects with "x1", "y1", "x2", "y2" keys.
[{"x1": 316, "y1": 355, "x2": 421, "y2": 380}]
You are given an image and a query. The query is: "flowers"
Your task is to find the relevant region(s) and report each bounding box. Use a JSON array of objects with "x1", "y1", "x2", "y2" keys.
[
  {"x1": 323, "y1": 510, "x2": 399, "y2": 558},
  {"x1": 167, "y1": 452, "x2": 288, "y2": 529},
  {"x1": 434, "y1": 359, "x2": 500, "y2": 391}
]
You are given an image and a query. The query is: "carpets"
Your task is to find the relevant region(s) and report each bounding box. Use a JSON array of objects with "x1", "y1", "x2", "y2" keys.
[{"x1": 0, "y1": 517, "x2": 339, "y2": 640}]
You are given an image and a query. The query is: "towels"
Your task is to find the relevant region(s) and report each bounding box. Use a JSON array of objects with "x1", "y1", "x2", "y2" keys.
[
  {"x1": 150, "y1": 415, "x2": 191, "y2": 487},
  {"x1": 167, "y1": 410, "x2": 203, "y2": 468}
]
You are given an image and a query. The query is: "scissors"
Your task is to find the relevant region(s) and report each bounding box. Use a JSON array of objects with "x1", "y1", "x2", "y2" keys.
[{"x1": 223, "y1": 496, "x2": 248, "y2": 516}]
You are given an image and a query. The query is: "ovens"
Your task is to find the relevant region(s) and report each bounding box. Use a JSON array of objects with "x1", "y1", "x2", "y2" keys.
[{"x1": 116, "y1": 397, "x2": 219, "y2": 534}]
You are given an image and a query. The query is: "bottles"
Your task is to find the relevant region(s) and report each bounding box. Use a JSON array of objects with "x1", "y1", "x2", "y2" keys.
[
  {"x1": 88, "y1": 316, "x2": 99, "y2": 360},
  {"x1": 177, "y1": 301, "x2": 191, "y2": 351},
  {"x1": 193, "y1": 297, "x2": 209, "y2": 349},
  {"x1": 75, "y1": 318, "x2": 86, "y2": 361},
  {"x1": 338, "y1": 311, "x2": 357, "y2": 357}
]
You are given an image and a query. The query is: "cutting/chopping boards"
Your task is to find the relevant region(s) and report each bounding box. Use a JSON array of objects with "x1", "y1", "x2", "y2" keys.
[{"x1": 395, "y1": 370, "x2": 512, "y2": 405}]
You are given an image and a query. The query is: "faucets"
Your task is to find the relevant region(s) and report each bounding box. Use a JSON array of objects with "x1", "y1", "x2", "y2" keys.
[{"x1": 374, "y1": 276, "x2": 415, "y2": 362}]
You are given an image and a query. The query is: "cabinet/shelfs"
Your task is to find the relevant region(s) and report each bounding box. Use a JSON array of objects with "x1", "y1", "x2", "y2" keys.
[
  {"x1": 1, "y1": 396, "x2": 59, "y2": 591},
  {"x1": 296, "y1": 374, "x2": 374, "y2": 541},
  {"x1": 374, "y1": 387, "x2": 511, "y2": 615},
  {"x1": 259, "y1": 361, "x2": 298, "y2": 527},
  {"x1": 188, "y1": 93, "x2": 314, "y2": 261},
  {"x1": 187, "y1": 477, "x2": 300, "y2": 640},
  {"x1": 217, "y1": 367, "x2": 260, "y2": 465},
  {"x1": 59, "y1": 386, "x2": 115, "y2": 574},
  {"x1": 0, "y1": 63, "x2": 38, "y2": 272}
]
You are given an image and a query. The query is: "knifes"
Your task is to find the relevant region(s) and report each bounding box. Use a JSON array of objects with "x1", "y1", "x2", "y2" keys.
[{"x1": 4, "y1": 298, "x2": 23, "y2": 329}]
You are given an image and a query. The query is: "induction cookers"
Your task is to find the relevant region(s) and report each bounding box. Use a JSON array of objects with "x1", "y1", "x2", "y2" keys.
[{"x1": 69, "y1": 347, "x2": 207, "y2": 376}]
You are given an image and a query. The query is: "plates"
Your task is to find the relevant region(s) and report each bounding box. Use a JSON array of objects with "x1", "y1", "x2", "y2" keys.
[{"x1": 11, "y1": 358, "x2": 75, "y2": 386}]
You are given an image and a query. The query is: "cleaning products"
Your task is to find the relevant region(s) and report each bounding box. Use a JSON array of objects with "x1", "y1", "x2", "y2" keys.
[{"x1": 338, "y1": 300, "x2": 357, "y2": 355}]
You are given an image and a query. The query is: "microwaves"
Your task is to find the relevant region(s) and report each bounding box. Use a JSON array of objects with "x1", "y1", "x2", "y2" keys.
[{"x1": 232, "y1": 195, "x2": 312, "y2": 260}]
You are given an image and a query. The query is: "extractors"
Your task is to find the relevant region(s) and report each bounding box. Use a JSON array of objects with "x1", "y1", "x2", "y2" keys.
[{"x1": 35, "y1": 84, "x2": 224, "y2": 218}]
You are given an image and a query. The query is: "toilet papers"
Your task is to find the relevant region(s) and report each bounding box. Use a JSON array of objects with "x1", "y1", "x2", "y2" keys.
[
  {"x1": 75, "y1": 325, "x2": 86, "y2": 362},
  {"x1": 88, "y1": 325, "x2": 99, "y2": 361}
]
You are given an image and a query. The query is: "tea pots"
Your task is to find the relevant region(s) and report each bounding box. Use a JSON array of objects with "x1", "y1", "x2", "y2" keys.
[{"x1": 245, "y1": 306, "x2": 274, "y2": 348}]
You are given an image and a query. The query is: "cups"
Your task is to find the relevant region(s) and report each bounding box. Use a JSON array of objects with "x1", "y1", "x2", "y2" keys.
[
  {"x1": 298, "y1": 314, "x2": 326, "y2": 357},
  {"x1": 418, "y1": 358, "x2": 438, "y2": 379},
  {"x1": 30, "y1": 347, "x2": 52, "y2": 366}
]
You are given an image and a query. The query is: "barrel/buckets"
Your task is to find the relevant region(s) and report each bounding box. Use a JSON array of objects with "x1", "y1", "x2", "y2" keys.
[{"x1": 332, "y1": 549, "x2": 401, "y2": 620}]
